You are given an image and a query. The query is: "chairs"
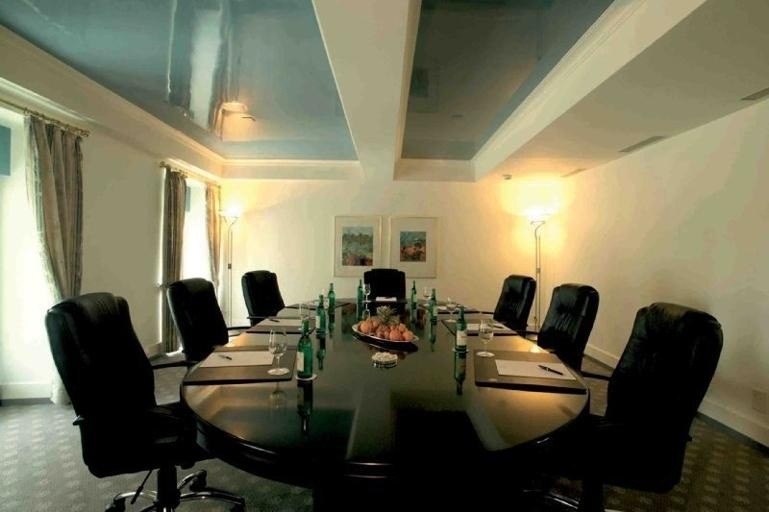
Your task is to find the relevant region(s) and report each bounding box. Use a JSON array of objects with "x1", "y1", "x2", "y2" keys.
[
  {"x1": 364, "y1": 269, "x2": 406, "y2": 300},
  {"x1": 241, "y1": 270, "x2": 285, "y2": 324},
  {"x1": 166, "y1": 278, "x2": 229, "y2": 360},
  {"x1": 520, "y1": 302, "x2": 724, "y2": 511},
  {"x1": 537, "y1": 283, "x2": 600, "y2": 370},
  {"x1": 45, "y1": 292, "x2": 246, "y2": 511},
  {"x1": 493, "y1": 274, "x2": 536, "y2": 338}
]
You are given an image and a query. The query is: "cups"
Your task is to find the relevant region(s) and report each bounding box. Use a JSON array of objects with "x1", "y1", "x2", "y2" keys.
[{"x1": 362, "y1": 284, "x2": 371, "y2": 302}]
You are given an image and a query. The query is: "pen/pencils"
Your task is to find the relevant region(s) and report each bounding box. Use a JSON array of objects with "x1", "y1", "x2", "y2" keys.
[
  {"x1": 219, "y1": 355, "x2": 232, "y2": 360},
  {"x1": 537, "y1": 365, "x2": 563, "y2": 376},
  {"x1": 269, "y1": 318, "x2": 279, "y2": 322},
  {"x1": 493, "y1": 325, "x2": 503, "y2": 329}
]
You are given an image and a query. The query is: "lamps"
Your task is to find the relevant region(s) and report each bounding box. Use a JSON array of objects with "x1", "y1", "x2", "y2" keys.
[
  {"x1": 527, "y1": 182, "x2": 555, "y2": 341},
  {"x1": 223, "y1": 197, "x2": 238, "y2": 335}
]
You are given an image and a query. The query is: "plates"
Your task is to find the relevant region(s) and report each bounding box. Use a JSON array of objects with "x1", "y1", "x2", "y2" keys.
[{"x1": 353, "y1": 318, "x2": 419, "y2": 343}]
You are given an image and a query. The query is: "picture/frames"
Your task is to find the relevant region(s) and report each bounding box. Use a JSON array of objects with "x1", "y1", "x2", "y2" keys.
[
  {"x1": 389, "y1": 216, "x2": 437, "y2": 279},
  {"x1": 334, "y1": 215, "x2": 382, "y2": 277}
]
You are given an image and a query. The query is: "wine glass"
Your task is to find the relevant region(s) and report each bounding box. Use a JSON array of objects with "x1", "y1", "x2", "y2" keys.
[
  {"x1": 298, "y1": 303, "x2": 310, "y2": 331},
  {"x1": 446, "y1": 297, "x2": 457, "y2": 324},
  {"x1": 362, "y1": 301, "x2": 370, "y2": 320},
  {"x1": 423, "y1": 286, "x2": 431, "y2": 306},
  {"x1": 477, "y1": 320, "x2": 495, "y2": 358},
  {"x1": 271, "y1": 380, "x2": 286, "y2": 413},
  {"x1": 268, "y1": 329, "x2": 290, "y2": 375}
]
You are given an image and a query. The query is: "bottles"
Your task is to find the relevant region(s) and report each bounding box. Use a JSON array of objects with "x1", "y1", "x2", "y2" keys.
[
  {"x1": 455, "y1": 353, "x2": 466, "y2": 398},
  {"x1": 356, "y1": 305, "x2": 366, "y2": 324},
  {"x1": 410, "y1": 280, "x2": 419, "y2": 306},
  {"x1": 357, "y1": 279, "x2": 364, "y2": 305},
  {"x1": 455, "y1": 307, "x2": 468, "y2": 352},
  {"x1": 313, "y1": 333, "x2": 327, "y2": 373},
  {"x1": 328, "y1": 310, "x2": 337, "y2": 340},
  {"x1": 428, "y1": 323, "x2": 439, "y2": 353},
  {"x1": 297, "y1": 321, "x2": 315, "y2": 379},
  {"x1": 429, "y1": 289, "x2": 439, "y2": 324},
  {"x1": 315, "y1": 295, "x2": 327, "y2": 334},
  {"x1": 409, "y1": 305, "x2": 418, "y2": 333},
  {"x1": 328, "y1": 283, "x2": 336, "y2": 317},
  {"x1": 296, "y1": 381, "x2": 314, "y2": 438}
]
